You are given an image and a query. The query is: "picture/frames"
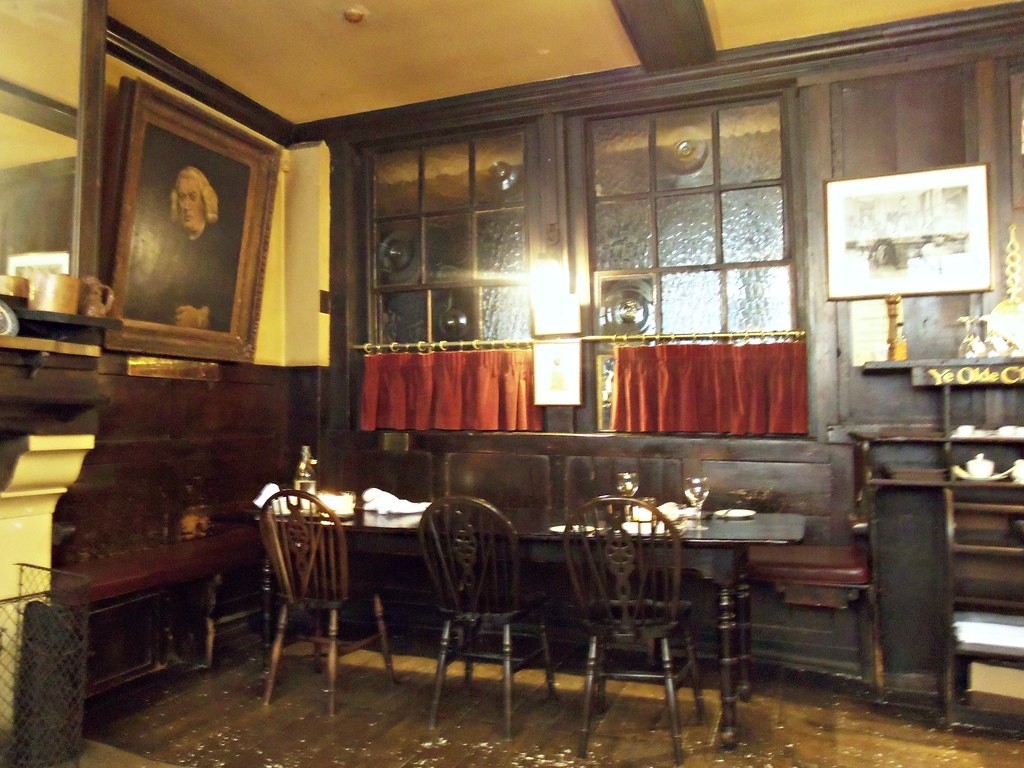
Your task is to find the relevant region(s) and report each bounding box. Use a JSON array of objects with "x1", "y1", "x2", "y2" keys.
[
  {"x1": 822, "y1": 162, "x2": 994, "y2": 302},
  {"x1": 103, "y1": 77, "x2": 282, "y2": 364},
  {"x1": 209, "y1": 292, "x2": 211, "y2": 294}
]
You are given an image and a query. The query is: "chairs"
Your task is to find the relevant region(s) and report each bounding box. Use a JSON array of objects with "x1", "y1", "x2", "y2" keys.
[
  {"x1": 258, "y1": 490, "x2": 395, "y2": 718},
  {"x1": 562, "y1": 496, "x2": 704, "y2": 766},
  {"x1": 418, "y1": 496, "x2": 557, "y2": 740}
]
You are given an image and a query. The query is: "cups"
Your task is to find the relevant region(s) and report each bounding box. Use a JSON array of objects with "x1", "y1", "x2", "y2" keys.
[
  {"x1": 632, "y1": 496, "x2": 656, "y2": 523},
  {"x1": 966, "y1": 459, "x2": 995, "y2": 477}
]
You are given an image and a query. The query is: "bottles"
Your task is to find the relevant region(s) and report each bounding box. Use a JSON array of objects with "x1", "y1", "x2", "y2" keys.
[{"x1": 293, "y1": 446, "x2": 317, "y2": 515}]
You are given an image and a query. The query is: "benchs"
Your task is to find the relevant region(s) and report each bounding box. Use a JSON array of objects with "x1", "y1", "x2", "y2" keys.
[{"x1": 316, "y1": 430, "x2": 874, "y2": 690}]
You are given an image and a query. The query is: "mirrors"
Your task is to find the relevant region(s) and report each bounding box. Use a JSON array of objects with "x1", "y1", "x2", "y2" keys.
[{"x1": 0, "y1": 0, "x2": 109, "y2": 300}]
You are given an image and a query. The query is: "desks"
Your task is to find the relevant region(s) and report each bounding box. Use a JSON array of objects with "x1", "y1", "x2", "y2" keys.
[{"x1": 222, "y1": 495, "x2": 806, "y2": 750}]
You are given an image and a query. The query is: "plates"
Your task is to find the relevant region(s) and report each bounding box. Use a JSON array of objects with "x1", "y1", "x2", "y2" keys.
[
  {"x1": 549, "y1": 525, "x2": 595, "y2": 536},
  {"x1": 714, "y1": 508, "x2": 757, "y2": 518}
]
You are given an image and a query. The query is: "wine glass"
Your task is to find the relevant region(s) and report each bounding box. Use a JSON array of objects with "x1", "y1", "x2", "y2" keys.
[
  {"x1": 683, "y1": 475, "x2": 710, "y2": 530},
  {"x1": 955, "y1": 313, "x2": 1024, "y2": 357},
  {"x1": 616, "y1": 471, "x2": 640, "y2": 521}
]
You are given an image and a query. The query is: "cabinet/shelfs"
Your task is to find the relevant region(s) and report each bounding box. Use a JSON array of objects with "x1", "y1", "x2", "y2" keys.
[{"x1": 848, "y1": 429, "x2": 1024, "y2": 738}]
